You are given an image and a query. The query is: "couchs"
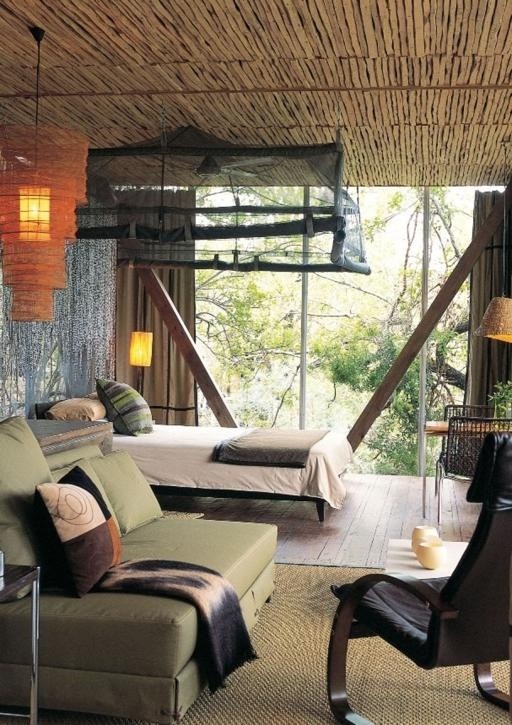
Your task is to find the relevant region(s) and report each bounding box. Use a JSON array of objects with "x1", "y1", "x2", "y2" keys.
[{"x1": 0, "y1": 415, "x2": 279, "y2": 725}]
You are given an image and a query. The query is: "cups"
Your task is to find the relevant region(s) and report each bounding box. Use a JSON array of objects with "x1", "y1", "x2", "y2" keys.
[{"x1": 416, "y1": 542, "x2": 447, "y2": 571}]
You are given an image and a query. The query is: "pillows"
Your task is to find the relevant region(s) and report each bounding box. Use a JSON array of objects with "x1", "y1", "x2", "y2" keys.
[
  {"x1": 0, "y1": 415, "x2": 165, "y2": 600},
  {"x1": 45, "y1": 377, "x2": 154, "y2": 436}
]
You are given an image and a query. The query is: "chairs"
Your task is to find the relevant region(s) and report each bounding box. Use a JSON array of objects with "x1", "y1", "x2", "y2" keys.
[
  {"x1": 435, "y1": 404, "x2": 512, "y2": 527},
  {"x1": 326, "y1": 432, "x2": 512, "y2": 725}
]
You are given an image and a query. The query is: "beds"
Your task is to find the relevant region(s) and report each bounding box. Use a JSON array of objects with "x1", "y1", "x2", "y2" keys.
[{"x1": 35, "y1": 397, "x2": 357, "y2": 521}]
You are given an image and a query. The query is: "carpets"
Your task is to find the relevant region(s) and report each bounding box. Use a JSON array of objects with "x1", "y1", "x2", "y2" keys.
[{"x1": 2, "y1": 562, "x2": 512, "y2": 724}]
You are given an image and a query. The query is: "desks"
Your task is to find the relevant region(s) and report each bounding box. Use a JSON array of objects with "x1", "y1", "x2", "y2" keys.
[
  {"x1": 1, "y1": 561, "x2": 40, "y2": 725},
  {"x1": 422, "y1": 418, "x2": 512, "y2": 519},
  {"x1": 384, "y1": 539, "x2": 484, "y2": 583}
]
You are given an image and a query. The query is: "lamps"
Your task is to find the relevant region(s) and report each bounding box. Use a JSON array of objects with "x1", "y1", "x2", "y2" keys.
[
  {"x1": 197, "y1": 155, "x2": 222, "y2": 178},
  {"x1": 474, "y1": 142, "x2": 512, "y2": 343},
  {"x1": 0, "y1": 26, "x2": 90, "y2": 323},
  {"x1": 129, "y1": 332, "x2": 154, "y2": 395}
]
